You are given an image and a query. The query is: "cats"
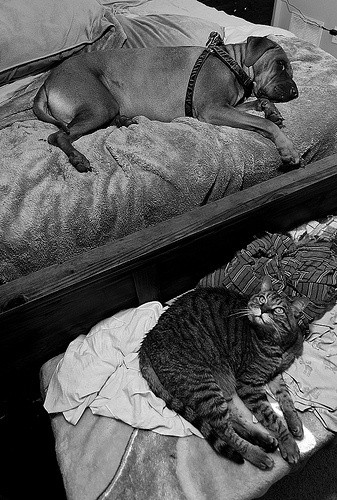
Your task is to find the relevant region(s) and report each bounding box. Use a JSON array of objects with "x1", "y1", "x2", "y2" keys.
[{"x1": 138, "y1": 273, "x2": 314, "y2": 474}]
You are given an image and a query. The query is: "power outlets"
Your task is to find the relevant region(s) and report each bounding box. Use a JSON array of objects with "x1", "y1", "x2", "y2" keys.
[{"x1": 332, "y1": 26, "x2": 337, "y2": 44}]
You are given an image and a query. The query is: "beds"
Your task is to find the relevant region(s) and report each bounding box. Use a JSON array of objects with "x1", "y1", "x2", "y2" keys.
[
  {"x1": 0, "y1": 215, "x2": 337, "y2": 500},
  {"x1": 0, "y1": 0, "x2": 337, "y2": 358}
]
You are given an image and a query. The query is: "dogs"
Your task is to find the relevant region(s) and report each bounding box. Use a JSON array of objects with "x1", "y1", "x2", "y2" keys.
[{"x1": 31, "y1": 29, "x2": 301, "y2": 175}]
